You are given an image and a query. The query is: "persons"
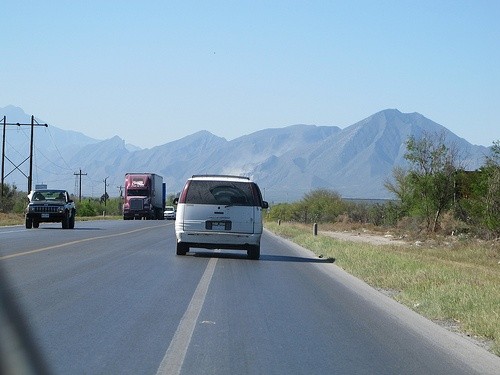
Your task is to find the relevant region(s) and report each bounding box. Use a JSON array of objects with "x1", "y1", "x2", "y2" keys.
[{"x1": 34, "y1": 193, "x2": 66, "y2": 200}]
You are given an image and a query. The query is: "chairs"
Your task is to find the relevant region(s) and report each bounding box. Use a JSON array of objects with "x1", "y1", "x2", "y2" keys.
[{"x1": 34, "y1": 194, "x2": 42, "y2": 200}]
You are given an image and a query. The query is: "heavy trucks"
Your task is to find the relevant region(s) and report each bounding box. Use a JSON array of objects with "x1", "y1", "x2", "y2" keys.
[{"x1": 121, "y1": 170, "x2": 166, "y2": 221}]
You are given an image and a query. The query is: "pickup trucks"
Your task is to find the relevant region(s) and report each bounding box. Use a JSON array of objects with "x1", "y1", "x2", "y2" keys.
[{"x1": 23, "y1": 189, "x2": 77, "y2": 229}]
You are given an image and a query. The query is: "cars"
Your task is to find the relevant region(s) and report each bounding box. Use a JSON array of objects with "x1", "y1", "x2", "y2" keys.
[
  {"x1": 173, "y1": 173, "x2": 269, "y2": 259},
  {"x1": 164, "y1": 206, "x2": 176, "y2": 220}
]
